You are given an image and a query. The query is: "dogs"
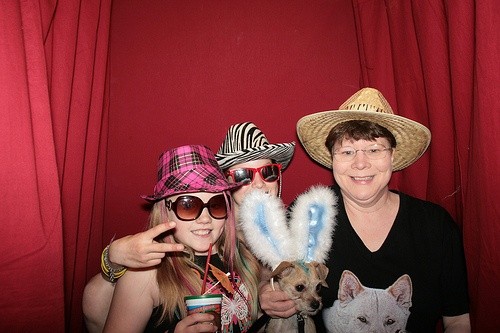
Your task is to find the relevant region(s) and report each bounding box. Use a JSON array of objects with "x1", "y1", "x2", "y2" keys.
[{"x1": 254, "y1": 258, "x2": 332, "y2": 333}]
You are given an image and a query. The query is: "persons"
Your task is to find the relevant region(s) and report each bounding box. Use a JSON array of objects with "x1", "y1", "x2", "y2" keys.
[
  {"x1": 81, "y1": 121, "x2": 295, "y2": 333},
  {"x1": 102, "y1": 144, "x2": 258, "y2": 333},
  {"x1": 259, "y1": 88, "x2": 472, "y2": 333}
]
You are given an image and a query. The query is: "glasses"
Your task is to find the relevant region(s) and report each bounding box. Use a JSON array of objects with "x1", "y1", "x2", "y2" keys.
[
  {"x1": 165, "y1": 194, "x2": 230, "y2": 221},
  {"x1": 223, "y1": 163, "x2": 283, "y2": 186},
  {"x1": 332, "y1": 144, "x2": 391, "y2": 162}
]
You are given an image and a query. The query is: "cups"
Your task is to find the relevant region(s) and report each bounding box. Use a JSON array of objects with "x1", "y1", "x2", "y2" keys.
[{"x1": 183, "y1": 293, "x2": 223, "y2": 333}]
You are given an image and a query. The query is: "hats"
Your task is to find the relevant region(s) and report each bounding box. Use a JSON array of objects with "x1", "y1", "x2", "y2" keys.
[
  {"x1": 297, "y1": 87, "x2": 432, "y2": 172},
  {"x1": 213, "y1": 121, "x2": 295, "y2": 171},
  {"x1": 139, "y1": 144, "x2": 244, "y2": 203}
]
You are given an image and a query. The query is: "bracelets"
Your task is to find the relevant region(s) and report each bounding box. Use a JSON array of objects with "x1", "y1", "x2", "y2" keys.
[{"x1": 100, "y1": 245, "x2": 127, "y2": 281}]
